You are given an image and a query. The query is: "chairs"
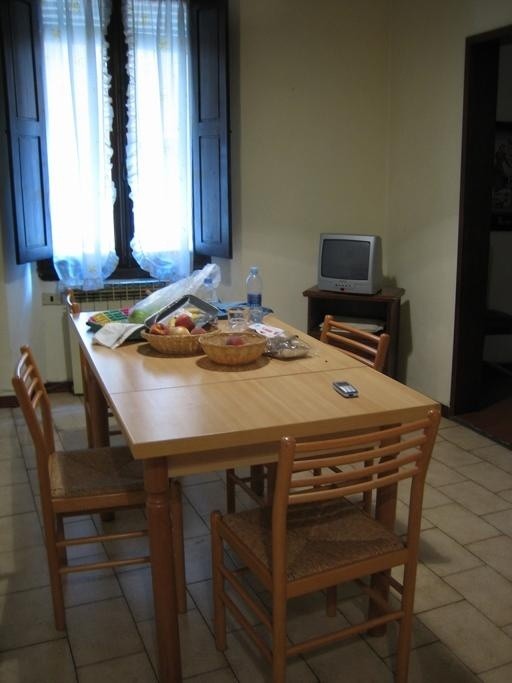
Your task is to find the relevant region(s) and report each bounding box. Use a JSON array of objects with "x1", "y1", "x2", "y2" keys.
[
  {"x1": 206, "y1": 407, "x2": 444, "y2": 682},
  {"x1": 225, "y1": 303, "x2": 391, "y2": 513},
  {"x1": 7, "y1": 339, "x2": 189, "y2": 629}
]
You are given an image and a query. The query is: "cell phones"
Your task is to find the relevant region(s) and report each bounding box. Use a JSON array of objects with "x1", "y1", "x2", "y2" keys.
[{"x1": 331, "y1": 381, "x2": 359, "y2": 397}]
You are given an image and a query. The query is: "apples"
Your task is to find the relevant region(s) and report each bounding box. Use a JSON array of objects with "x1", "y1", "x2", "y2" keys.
[
  {"x1": 226, "y1": 336, "x2": 245, "y2": 345},
  {"x1": 149, "y1": 315, "x2": 206, "y2": 336}
]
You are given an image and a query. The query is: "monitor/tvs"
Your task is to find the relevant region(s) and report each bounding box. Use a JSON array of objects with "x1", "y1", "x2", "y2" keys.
[{"x1": 317, "y1": 233, "x2": 383, "y2": 295}]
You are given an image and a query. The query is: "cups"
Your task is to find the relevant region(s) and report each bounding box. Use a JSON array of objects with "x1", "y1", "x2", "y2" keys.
[{"x1": 227, "y1": 304, "x2": 247, "y2": 332}]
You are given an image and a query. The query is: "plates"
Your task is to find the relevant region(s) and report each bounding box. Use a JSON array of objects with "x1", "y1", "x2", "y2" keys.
[{"x1": 268, "y1": 340, "x2": 310, "y2": 357}]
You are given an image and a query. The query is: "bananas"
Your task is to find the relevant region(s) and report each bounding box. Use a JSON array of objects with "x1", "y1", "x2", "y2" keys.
[{"x1": 185, "y1": 307, "x2": 206, "y2": 320}]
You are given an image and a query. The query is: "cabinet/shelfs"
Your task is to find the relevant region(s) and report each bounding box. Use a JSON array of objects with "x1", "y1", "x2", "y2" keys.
[{"x1": 303, "y1": 285, "x2": 406, "y2": 380}]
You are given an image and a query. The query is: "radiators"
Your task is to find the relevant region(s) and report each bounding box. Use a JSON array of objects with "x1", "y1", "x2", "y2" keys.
[{"x1": 59, "y1": 278, "x2": 177, "y2": 396}]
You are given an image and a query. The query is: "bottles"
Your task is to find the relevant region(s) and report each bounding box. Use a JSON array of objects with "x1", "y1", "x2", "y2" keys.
[
  {"x1": 202, "y1": 278, "x2": 219, "y2": 308},
  {"x1": 245, "y1": 266, "x2": 264, "y2": 323}
]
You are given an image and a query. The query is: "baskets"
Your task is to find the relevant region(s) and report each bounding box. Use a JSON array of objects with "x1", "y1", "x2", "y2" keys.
[
  {"x1": 140, "y1": 324, "x2": 222, "y2": 356},
  {"x1": 198, "y1": 331, "x2": 269, "y2": 366}
]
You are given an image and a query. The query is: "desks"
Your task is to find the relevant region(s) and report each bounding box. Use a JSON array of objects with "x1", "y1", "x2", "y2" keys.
[{"x1": 68, "y1": 301, "x2": 446, "y2": 681}]
